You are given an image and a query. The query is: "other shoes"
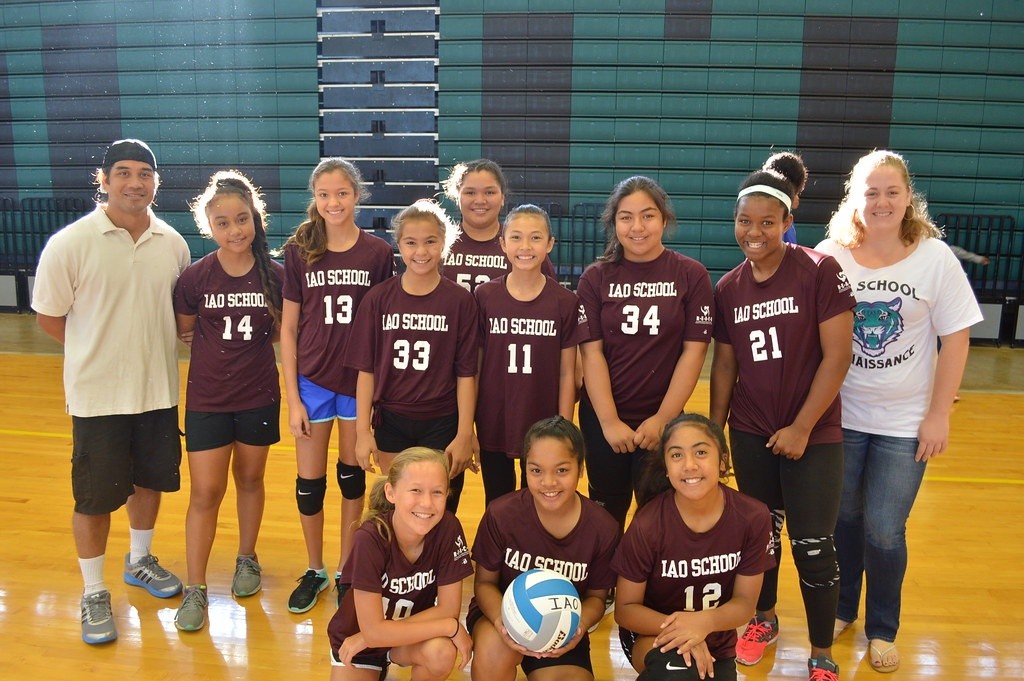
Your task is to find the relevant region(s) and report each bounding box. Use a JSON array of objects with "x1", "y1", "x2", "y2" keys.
[{"x1": 589, "y1": 594, "x2": 616, "y2": 634}]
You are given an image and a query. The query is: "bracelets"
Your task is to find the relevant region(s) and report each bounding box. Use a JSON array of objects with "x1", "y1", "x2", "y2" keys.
[{"x1": 448, "y1": 617, "x2": 459, "y2": 638}]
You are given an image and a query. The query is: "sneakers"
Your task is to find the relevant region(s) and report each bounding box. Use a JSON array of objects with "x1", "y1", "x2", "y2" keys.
[
  {"x1": 174, "y1": 580, "x2": 208, "y2": 631},
  {"x1": 808, "y1": 655, "x2": 839, "y2": 681},
  {"x1": 80, "y1": 585, "x2": 118, "y2": 644},
  {"x1": 288, "y1": 567, "x2": 330, "y2": 614},
  {"x1": 231, "y1": 556, "x2": 263, "y2": 596},
  {"x1": 735, "y1": 613, "x2": 779, "y2": 665},
  {"x1": 334, "y1": 571, "x2": 348, "y2": 610},
  {"x1": 123, "y1": 552, "x2": 183, "y2": 597}
]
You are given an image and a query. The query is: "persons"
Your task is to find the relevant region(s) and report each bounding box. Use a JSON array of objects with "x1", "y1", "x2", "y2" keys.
[
  {"x1": 708, "y1": 149, "x2": 858, "y2": 681},
  {"x1": 327, "y1": 447, "x2": 474, "y2": 681},
  {"x1": 438, "y1": 158, "x2": 558, "y2": 293},
  {"x1": 343, "y1": 195, "x2": 479, "y2": 515},
  {"x1": 466, "y1": 414, "x2": 621, "y2": 681},
  {"x1": 173, "y1": 169, "x2": 285, "y2": 630},
  {"x1": 269, "y1": 156, "x2": 393, "y2": 614},
  {"x1": 575, "y1": 174, "x2": 715, "y2": 632},
  {"x1": 950, "y1": 247, "x2": 991, "y2": 404},
  {"x1": 608, "y1": 417, "x2": 777, "y2": 681},
  {"x1": 457, "y1": 203, "x2": 591, "y2": 518},
  {"x1": 30, "y1": 138, "x2": 191, "y2": 646},
  {"x1": 810, "y1": 147, "x2": 985, "y2": 675}
]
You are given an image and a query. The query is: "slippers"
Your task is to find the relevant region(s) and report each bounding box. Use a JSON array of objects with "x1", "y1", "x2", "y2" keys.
[
  {"x1": 869, "y1": 633, "x2": 898, "y2": 673},
  {"x1": 832, "y1": 615, "x2": 851, "y2": 641}
]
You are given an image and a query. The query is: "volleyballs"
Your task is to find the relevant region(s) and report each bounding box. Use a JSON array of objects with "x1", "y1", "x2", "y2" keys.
[{"x1": 501, "y1": 569, "x2": 582, "y2": 652}]
